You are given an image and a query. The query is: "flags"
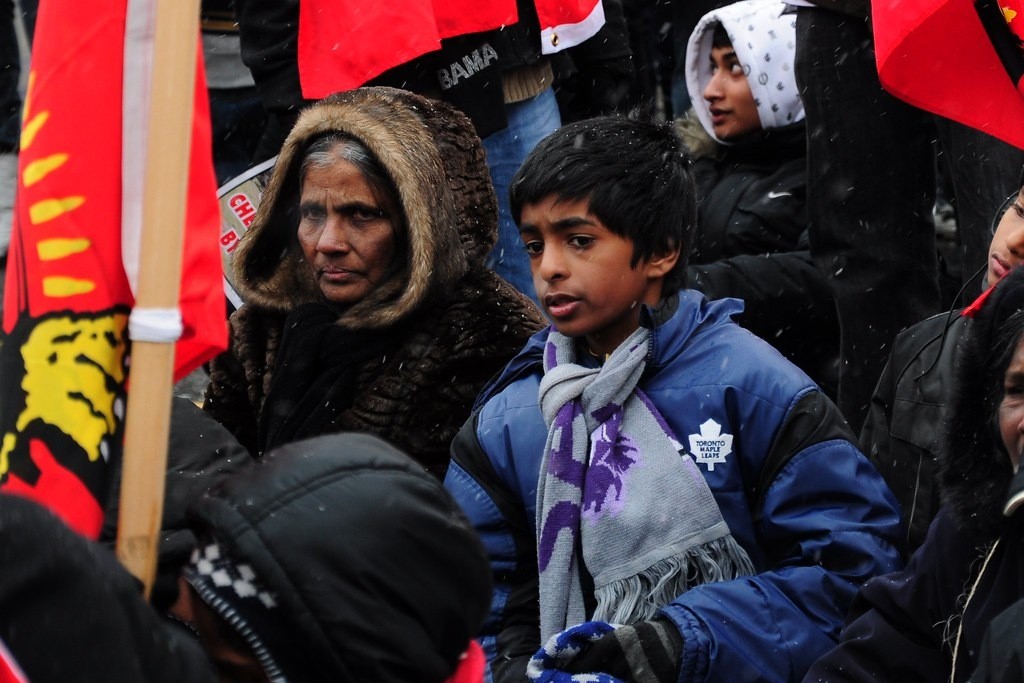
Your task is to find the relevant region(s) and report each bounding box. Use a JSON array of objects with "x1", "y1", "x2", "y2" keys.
[
  {"x1": 0, "y1": 0, "x2": 232, "y2": 547},
  {"x1": 871, "y1": 0, "x2": 1024, "y2": 151}
]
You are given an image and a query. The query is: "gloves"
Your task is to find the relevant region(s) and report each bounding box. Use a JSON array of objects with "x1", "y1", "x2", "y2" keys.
[{"x1": 565, "y1": 620, "x2": 684, "y2": 683}]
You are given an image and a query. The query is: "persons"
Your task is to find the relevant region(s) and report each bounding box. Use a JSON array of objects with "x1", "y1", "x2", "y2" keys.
[{"x1": 0, "y1": 0, "x2": 1024, "y2": 683}]
